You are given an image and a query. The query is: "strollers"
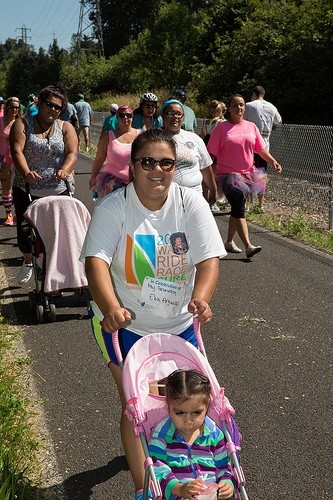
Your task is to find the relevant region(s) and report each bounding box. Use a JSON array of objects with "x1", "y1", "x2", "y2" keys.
[
  {"x1": 25, "y1": 173, "x2": 99, "y2": 315},
  {"x1": 112, "y1": 310, "x2": 251, "y2": 500}
]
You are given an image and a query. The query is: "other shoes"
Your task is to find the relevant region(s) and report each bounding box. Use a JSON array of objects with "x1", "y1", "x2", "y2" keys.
[
  {"x1": 85, "y1": 147, "x2": 88, "y2": 152},
  {"x1": 78, "y1": 146, "x2": 80, "y2": 151}
]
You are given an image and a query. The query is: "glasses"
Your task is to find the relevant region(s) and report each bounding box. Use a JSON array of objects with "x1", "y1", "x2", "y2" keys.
[
  {"x1": 42, "y1": 100, "x2": 64, "y2": 111},
  {"x1": 7, "y1": 105, "x2": 20, "y2": 110},
  {"x1": 134, "y1": 157, "x2": 176, "y2": 172},
  {"x1": 117, "y1": 113, "x2": 132, "y2": 119},
  {"x1": 144, "y1": 105, "x2": 156, "y2": 109},
  {"x1": 163, "y1": 111, "x2": 184, "y2": 120}
]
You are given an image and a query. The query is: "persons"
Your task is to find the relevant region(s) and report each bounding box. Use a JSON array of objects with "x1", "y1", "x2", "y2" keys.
[
  {"x1": 80, "y1": 128, "x2": 227, "y2": 500},
  {"x1": 157, "y1": 90, "x2": 198, "y2": 132},
  {"x1": 0, "y1": 94, "x2": 78, "y2": 226},
  {"x1": 200, "y1": 100, "x2": 230, "y2": 211},
  {"x1": 9, "y1": 86, "x2": 80, "y2": 284},
  {"x1": 99, "y1": 103, "x2": 119, "y2": 138},
  {"x1": 158, "y1": 98, "x2": 217, "y2": 207},
  {"x1": 244, "y1": 86, "x2": 282, "y2": 212},
  {"x1": 89, "y1": 106, "x2": 145, "y2": 202},
  {"x1": 207, "y1": 93, "x2": 282, "y2": 258},
  {"x1": 148, "y1": 369, "x2": 238, "y2": 500},
  {"x1": 130, "y1": 93, "x2": 164, "y2": 128},
  {"x1": 74, "y1": 93, "x2": 93, "y2": 150}
]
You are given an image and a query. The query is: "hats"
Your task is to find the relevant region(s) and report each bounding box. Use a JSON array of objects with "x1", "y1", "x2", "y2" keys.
[
  {"x1": 167, "y1": 90, "x2": 187, "y2": 101},
  {"x1": 110, "y1": 103, "x2": 119, "y2": 112}
]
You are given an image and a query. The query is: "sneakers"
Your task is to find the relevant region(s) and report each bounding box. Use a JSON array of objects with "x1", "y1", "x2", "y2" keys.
[
  {"x1": 5, "y1": 212, "x2": 15, "y2": 225},
  {"x1": 224, "y1": 242, "x2": 242, "y2": 252},
  {"x1": 247, "y1": 246, "x2": 262, "y2": 258},
  {"x1": 216, "y1": 197, "x2": 225, "y2": 203},
  {"x1": 16, "y1": 261, "x2": 33, "y2": 283},
  {"x1": 254, "y1": 205, "x2": 264, "y2": 212},
  {"x1": 211, "y1": 203, "x2": 220, "y2": 212}
]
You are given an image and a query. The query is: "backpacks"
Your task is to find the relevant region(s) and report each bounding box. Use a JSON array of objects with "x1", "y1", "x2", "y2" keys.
[{"x1": 203, "y1": 119, "x2": 223, "y2": 165}]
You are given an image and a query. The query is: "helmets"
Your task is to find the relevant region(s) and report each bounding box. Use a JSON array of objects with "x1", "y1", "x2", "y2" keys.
[{"x1": 139, "y1": 93, "x2": 158, "y2": 103}]
